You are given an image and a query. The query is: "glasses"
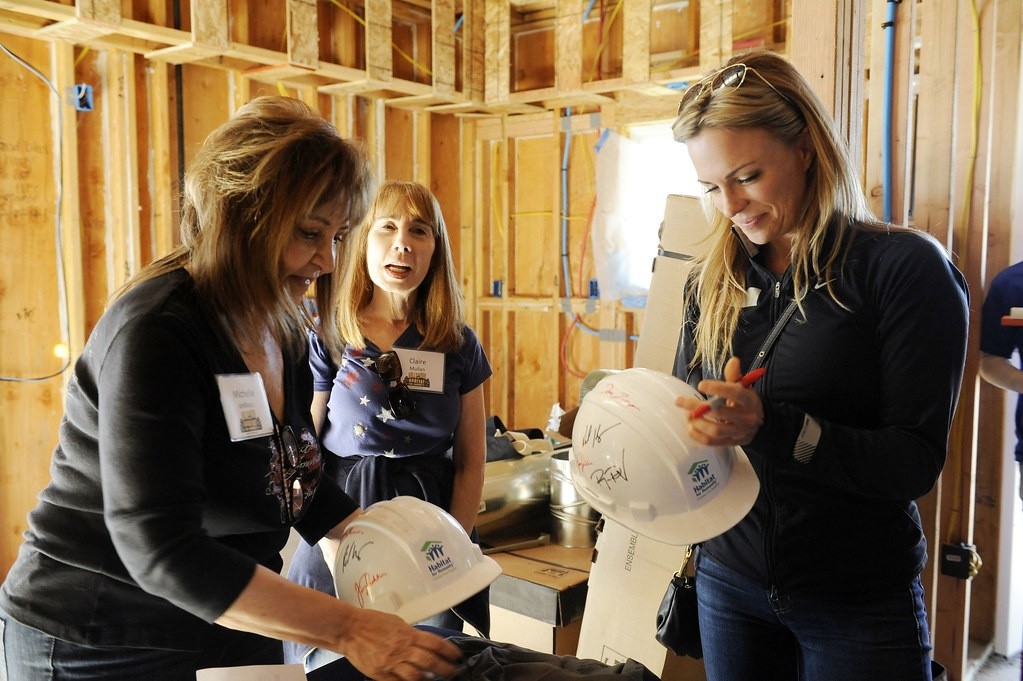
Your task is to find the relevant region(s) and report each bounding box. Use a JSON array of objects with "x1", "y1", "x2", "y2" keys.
[
  {"x1": 280, "y1": 426, "x2": 305, "y2": 520},
  {"x1": 374, "y1": 350, "x2": 417, "y2": 420},
  {"x1": 678, "y1": 63, "x2": 805, "y2": 120}
]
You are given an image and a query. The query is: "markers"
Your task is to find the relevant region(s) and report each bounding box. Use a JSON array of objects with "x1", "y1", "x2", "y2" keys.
[{"x1": 687, "y1": 368, "x2": 767, "y2": 422}]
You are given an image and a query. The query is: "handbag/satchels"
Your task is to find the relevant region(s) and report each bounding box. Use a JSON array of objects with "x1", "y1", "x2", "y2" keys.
[{"x1": 655, "y1": 571, "x2": 704, "y2": 662}]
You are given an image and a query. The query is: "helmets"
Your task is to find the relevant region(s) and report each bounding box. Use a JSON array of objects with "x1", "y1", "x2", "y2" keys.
[
  {"x1": 568, "y1": 368, "x2": 760, "y2": 545},
  {"x1": 333, "y1": 496, "x2": 503, "y2": 625}
]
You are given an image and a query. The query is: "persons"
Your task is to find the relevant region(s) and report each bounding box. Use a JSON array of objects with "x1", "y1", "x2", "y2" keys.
[
  {"x1": 0, "y1": 95, "x2": 465, "y2": 681},
  {"x1": 669, "y1": 48, "x2": 970, "y2": 681},
  {"x1": 284, "y1": 180, "x2": 492, "y2": 674},
  {"x1": 977, "y1": 263, "x2": 1023, "y2": 497}
]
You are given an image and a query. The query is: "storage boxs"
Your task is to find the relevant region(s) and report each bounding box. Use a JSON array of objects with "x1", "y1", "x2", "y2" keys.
[
  {"x1": 457, "y1": 533, "x2": 594, "y2": 657},
  {"x1": 575, "y1": 515, "x2": 706, "y2": 681}
]
